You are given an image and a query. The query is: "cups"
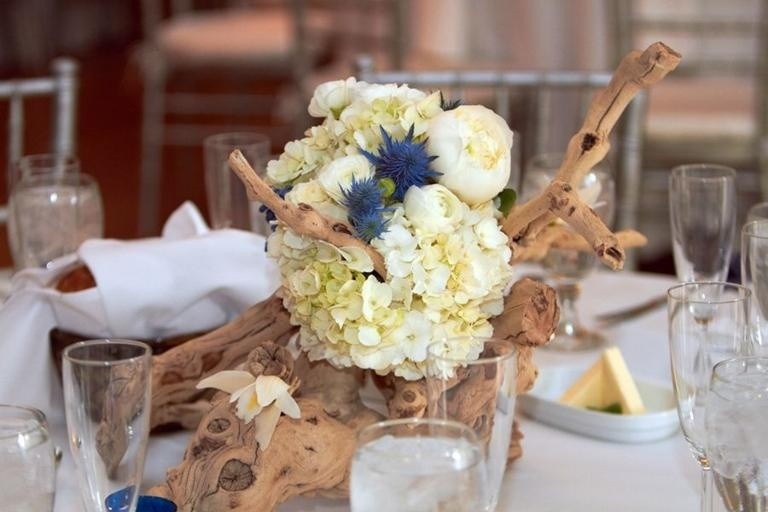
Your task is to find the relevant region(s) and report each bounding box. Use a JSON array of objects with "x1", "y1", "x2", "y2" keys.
[
  {"x1": 201, "y1": 130, "x2": 271, "y2": 233},
  {"x1": 2, "y1": 403, "x2": 50, "y2": 512},
  {"x1": 422, "y1": 335, "x2": 521, "y2": 511},
  {"x1": 5, "y1": 150, "x2": 105, "y2": 273},
  {"x1": 57, "y1": 340, "x2": 154, "y2": 512},
  {"x1": 348, "y1": 418, "x2": 488, "y2": 510}
]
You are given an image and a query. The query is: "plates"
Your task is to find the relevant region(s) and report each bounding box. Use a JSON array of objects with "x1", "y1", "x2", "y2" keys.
[{"x1": 525, "y1": 366, "x2": 679, "y2": 443}]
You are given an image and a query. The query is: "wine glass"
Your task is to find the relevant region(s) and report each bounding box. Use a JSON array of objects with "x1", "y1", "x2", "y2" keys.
[
  {"x1": 521, "y1": 149, "x2": 622, "y2": 354},
  {"x1": 664, "y1": 162, "x2": 768, "y2": 512}
]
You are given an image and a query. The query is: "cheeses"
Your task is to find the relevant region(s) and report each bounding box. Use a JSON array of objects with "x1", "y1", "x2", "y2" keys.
[{"x1": 559, "y1": 346, "x2": 645, "y2": 416}]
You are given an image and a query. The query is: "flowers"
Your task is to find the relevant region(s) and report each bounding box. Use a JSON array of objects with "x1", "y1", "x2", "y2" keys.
[{"x1": 195, "y1": 76, "x2": 524, "y2": 452}]
